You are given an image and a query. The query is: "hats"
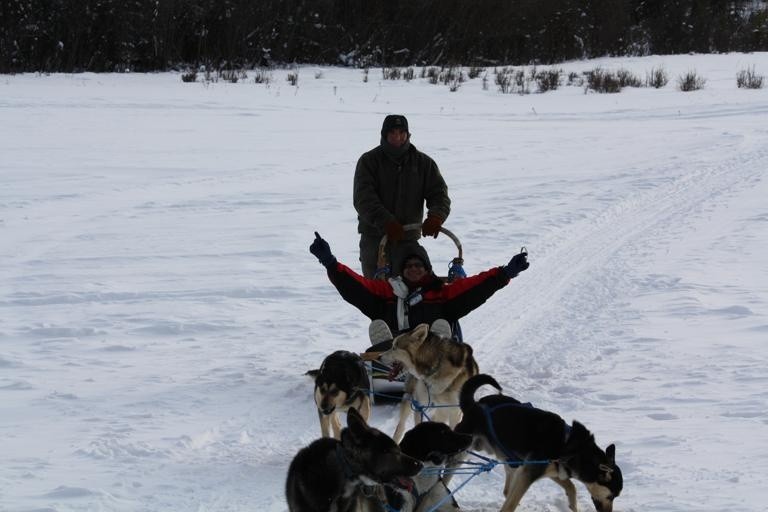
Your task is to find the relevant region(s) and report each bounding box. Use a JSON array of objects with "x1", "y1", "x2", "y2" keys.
[{"x1": 381, "y1": 114, "x2": 408, "y2": 139}]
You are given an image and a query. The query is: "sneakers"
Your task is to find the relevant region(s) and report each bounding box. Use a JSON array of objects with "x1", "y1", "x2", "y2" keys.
[
  {"x1": 430, "y1": 319, "x2": 452, "y2": 340},
  {"x1": 368, "y1": 319, "x2": 394, "y2": 346}
]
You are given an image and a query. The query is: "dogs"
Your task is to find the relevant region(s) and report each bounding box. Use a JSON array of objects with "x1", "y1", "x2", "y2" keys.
[
  {"x1": 378, "y1": 421, "x2": 473, "y2": 512},
  {"x1": 377, "y1": 322, "x2": 479, "y2": 446},
  {"x1": 285, "y1": 406, "x2": 424, "y2": 512},
  {"x1": 445, "y1": 373, "x2": 624, "y2": 512},
  {"x1": 301, "y1": 349, "x2": 372, "y2": 441}
]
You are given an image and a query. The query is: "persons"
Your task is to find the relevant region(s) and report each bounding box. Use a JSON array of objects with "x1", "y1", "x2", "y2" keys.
[
  {"x1": 310, "y1": 230, "x2": 530, "y2": 349},
  {"x1": 353, "y1": 114, "x2": 453, "y2": 277}
]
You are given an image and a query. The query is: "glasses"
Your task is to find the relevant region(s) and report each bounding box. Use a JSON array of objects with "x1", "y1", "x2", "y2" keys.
[{"x1": 403, "y1": 262, "x2": 424, "y2": 269}]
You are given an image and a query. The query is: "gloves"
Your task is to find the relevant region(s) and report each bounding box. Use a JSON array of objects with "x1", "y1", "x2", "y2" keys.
[
  {"x1": 309, "y1": 231, "x2": 334, "y2": 266},
  {"x1": 503, "y1": 252, "x2": 530, "y2": 279},
  {"x1": 385, "y1": 219, "x2": 407, "y2": 242},
  {"x1": 421, "y1": 215, "x2": 444, "y2": 239}
]
